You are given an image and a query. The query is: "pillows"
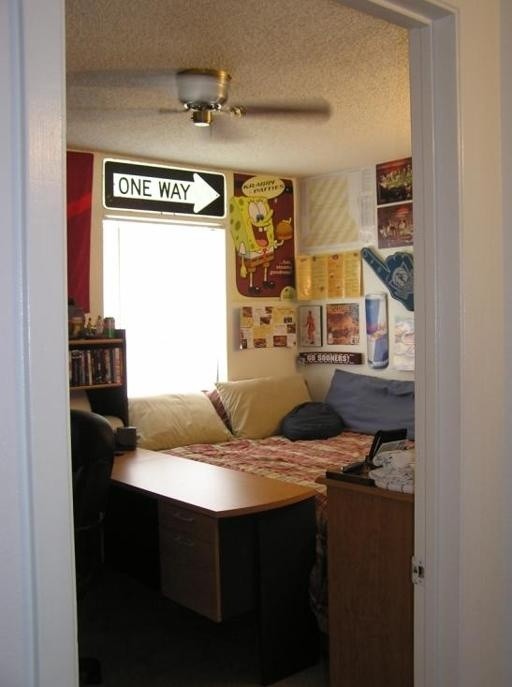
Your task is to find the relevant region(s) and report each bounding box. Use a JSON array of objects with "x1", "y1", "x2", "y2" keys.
[{"x1": 129, "y1": 372, "x2": 415, "y2": 450}]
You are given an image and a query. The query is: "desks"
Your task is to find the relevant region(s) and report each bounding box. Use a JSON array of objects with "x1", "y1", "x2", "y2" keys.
[
  {"x1": 108, "y1": 437, "x2": 319, "y2": 624},
  {"x1": 314, "y1": 471, "x2": 414, "y2": 686}
]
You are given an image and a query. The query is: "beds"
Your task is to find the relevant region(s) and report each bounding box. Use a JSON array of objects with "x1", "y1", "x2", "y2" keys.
[{"x1": 131, "y1": 370, "x2": 418, "y2": 633}]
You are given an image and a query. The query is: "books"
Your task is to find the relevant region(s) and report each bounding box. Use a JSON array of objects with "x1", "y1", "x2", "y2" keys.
[{"x1": 70, "y1": 348, "x2": 123, "y2": 386}]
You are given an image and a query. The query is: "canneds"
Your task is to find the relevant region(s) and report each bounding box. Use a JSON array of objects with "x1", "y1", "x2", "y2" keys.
[{"x1": 103, "y1": 317, "x2": 115, "y2": 338}]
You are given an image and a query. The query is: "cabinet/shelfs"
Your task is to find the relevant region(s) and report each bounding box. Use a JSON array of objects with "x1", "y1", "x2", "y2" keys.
[{"x1": 67, "y1": 327, "x2": 126, "y2": 391}]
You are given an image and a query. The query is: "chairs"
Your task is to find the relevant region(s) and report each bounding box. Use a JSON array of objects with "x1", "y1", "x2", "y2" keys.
[{"x1": 71, "y1": 409, "x2": 116, "y2": 564}]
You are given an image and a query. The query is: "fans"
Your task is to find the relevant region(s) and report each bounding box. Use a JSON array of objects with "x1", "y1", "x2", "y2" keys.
[{"x1": 74, "y1": 66, "x2": 333, "y2": 128}]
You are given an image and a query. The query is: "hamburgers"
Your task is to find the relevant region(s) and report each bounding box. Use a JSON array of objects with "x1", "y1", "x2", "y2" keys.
[{"x1": 277, "y1": 223, "x2": 293, "y2": 240}]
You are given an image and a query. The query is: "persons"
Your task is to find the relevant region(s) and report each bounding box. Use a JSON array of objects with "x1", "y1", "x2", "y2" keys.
[{"x1": 302, "y1": 311, "x2": 317, "y2": 346}]
